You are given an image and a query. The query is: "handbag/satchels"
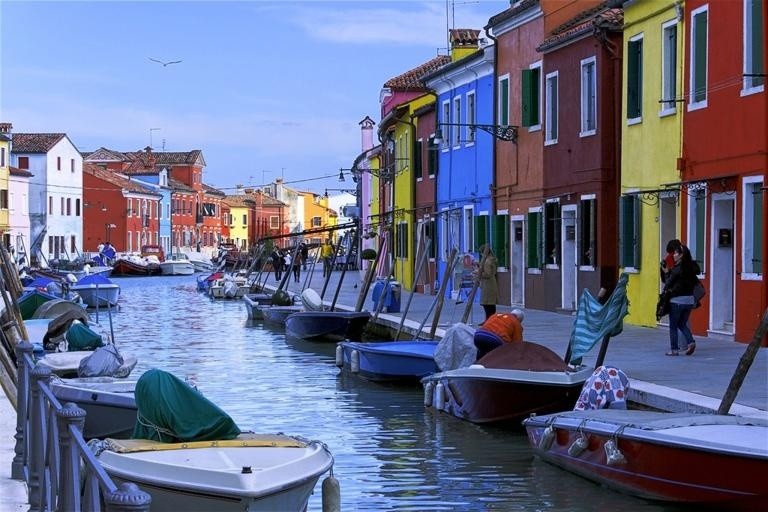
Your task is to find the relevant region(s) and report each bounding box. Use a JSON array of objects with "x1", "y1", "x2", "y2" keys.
[
  {"x1": 693, "y1": 281, "x2": 705, "y2": 301},
  {"x1": 656, "y1": 290, "x2": 671, "y2": 316}
]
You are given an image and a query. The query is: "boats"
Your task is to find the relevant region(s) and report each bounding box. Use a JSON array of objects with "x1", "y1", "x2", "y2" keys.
[
  {"x1": 243, "y1": 290, "x2": 301, "y2": 324},
  {"x1": 335, "y1": 339, "x2": 441, "y2": 383},
  {"x1": 196, "y1": 270, "x2": 251, "y2": 298},
  {"x1": 82, "y1": 368, "x2": 334, "y2": 512},
  {"x1": 17, "y1": 265, "x2": 138, "y2": 377},
  {"x1": 421, "y1": 339, "x2": 595, "y2": 424},
  {"x1": 113, "y1": 245, "x2": 212, "y2": 278},
  {"x1": 48, "y1": 343, "x2": 197, "y2": 438},
  {"x1": 282, "y1": 308, "x2": 370, "y2": 341},
  {"x1": 521, "y1": 409, "x2": 768, "y2": 506}
]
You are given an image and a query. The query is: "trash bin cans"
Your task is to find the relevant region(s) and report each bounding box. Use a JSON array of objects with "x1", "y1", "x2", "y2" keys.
[{"x1": 373, "y1": 281, "x2": 401, "y2": 313}]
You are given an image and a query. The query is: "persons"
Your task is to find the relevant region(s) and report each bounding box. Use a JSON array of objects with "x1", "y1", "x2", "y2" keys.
[
  {"x1": 294, "y1": 249, "x2": 304, "y2": 283},
  {"x1": 320, "y1": 239, "x2": 334, "y2": 278},
  {"x1": 301, "y1": 242, "x2": 309, "y2": 272},
  {"x1": 473, "y1": 309, "x2": 524, "y2": 363},
  {"x1": 97, "y1": 241, "x2": 117, "y2": 267},
  {"x1": 285, "y1": 251, "x2": 292, "y2": 271},
  {"x1": 661, "y1": 245, "x2": 697, "y2": 356},
  {"x1": 663, "y1": 239, "x2": 694, "y2": 352},
  {"x1": 272, "y1": 245, "x2": 284, "y2": 281},
  {"x1": 472, "y1": 242, "x2": 500, "y2": 326}
]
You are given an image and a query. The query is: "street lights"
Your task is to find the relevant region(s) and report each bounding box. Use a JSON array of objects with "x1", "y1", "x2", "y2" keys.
[{"x1": 338, "y1": 166, "x2": 353, "y2": 182}]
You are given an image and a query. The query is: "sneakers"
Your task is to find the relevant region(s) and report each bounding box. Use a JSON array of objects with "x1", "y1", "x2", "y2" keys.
[
  {"x1": 686, "y1": 343, "x2": 696, "y2": 354},
  {"x1": 665, "y1": 351, "x2": 678, "y2": 356}
]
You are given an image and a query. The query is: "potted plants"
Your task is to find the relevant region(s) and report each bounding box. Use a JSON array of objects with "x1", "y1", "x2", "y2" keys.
[{"x1": 360, "y1": 249, "x2": 377, "y2": 269}]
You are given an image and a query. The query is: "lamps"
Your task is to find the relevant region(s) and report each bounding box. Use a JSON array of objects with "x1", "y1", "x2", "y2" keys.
[
  {"x1": 324, "y1": 188, "x2": 360, "y2": 198},
  {"x1": 174, "y1": 209, "x2": 191, "y2": 216},
  {"x1": 338, "y1": 168, "x2": 379, "y2": 182},
  {"x1": 83, "y1": 203, "x2": 108, "y2": 211},
  {"x1": 433, "y1": 123, "x2": 519, "y2": 144}
]
